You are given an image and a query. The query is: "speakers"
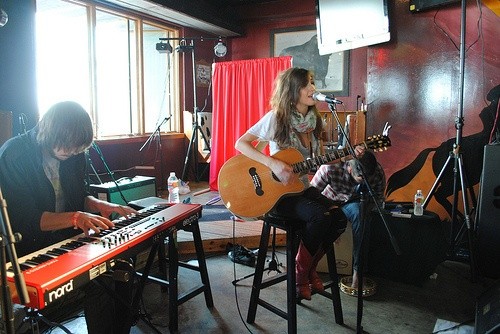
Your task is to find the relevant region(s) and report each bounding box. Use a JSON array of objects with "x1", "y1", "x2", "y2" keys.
[
  {"x1": 474, "y1": 144, "x2": 500, "y2": 280},
  {"x1": 474, "y1": 282, "x2": 500, "y2": 334}
]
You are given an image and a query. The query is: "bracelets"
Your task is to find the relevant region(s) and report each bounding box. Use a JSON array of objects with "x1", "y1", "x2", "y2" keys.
[{"x1": 72, "y1": 210, "x2": 81, "y2": 229}]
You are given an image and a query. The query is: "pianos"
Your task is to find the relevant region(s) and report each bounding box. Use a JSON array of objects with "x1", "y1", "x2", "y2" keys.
[{"x1": 0, "y1": 202, "x2": 214, "y2": 334}]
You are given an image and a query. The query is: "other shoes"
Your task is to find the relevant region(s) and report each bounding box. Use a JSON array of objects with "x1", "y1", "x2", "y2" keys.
[{"x1": 228, "y1": 251, "x2": 255, "y2": 267}]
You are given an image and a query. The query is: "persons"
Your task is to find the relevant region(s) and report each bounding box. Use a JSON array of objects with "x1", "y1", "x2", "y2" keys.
[
  {"x1": 235, "y1": 67, "x2": 365, "y2": 302},
  {"x1": 0, "y1": 101, "x2": 139, "y2": 334},
  {"x1": 310, "y1": 150, "x2": 387, "y2": 289}
]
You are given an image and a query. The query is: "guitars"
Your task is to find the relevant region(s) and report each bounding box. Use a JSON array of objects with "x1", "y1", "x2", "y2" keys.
[{"x1": 217, "y1": 119, "x2": 393, "y2": 221}]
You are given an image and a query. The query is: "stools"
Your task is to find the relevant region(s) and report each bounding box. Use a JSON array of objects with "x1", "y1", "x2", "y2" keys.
[
  {"x1": 131, "y1": 196, "x2": 215, "y2": 334},
  {"x1": 247, "y1": 214, "x2": 344, "y2": 334}
]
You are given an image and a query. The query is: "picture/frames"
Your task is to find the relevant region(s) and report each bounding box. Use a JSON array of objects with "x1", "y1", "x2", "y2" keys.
[{"x1": 269, "y1": 25, "x2": 349, "y2": 97}]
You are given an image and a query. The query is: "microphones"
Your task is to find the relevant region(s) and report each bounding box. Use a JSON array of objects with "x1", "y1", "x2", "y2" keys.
[
  {"x1": 312, "y1": 91, "x2": 344, "y2": 104},
  {"x1": 166, "y1": 114, "x2": 172, "y2": 120}
]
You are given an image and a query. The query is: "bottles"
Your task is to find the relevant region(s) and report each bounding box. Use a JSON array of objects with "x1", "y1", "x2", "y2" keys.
[
  {"x1": 168, "y1": 172, "x2": 180, "y2": 203},
  {"x1": 414, "y1": 190, "x2": 423, "y2": 216}
]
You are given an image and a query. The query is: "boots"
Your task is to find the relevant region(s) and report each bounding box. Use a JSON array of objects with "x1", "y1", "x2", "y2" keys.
[
  {"x1": 309, "y1": 246, "x2": 324, "y2": 295},
  {"x1": 295, "y1": 239, "x2": 314, "y2": 301}
]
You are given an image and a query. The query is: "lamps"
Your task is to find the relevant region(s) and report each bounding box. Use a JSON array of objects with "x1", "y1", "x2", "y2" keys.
[
  {"x1": 156, "y1": 39, "x2": 172, "y2": 53},
  {"x1": 214, "y1": 36, "x2": 227, "y2": 57},
  {"x1": 176, "y1": 39, "x2": 193, "y2": 53}
]
